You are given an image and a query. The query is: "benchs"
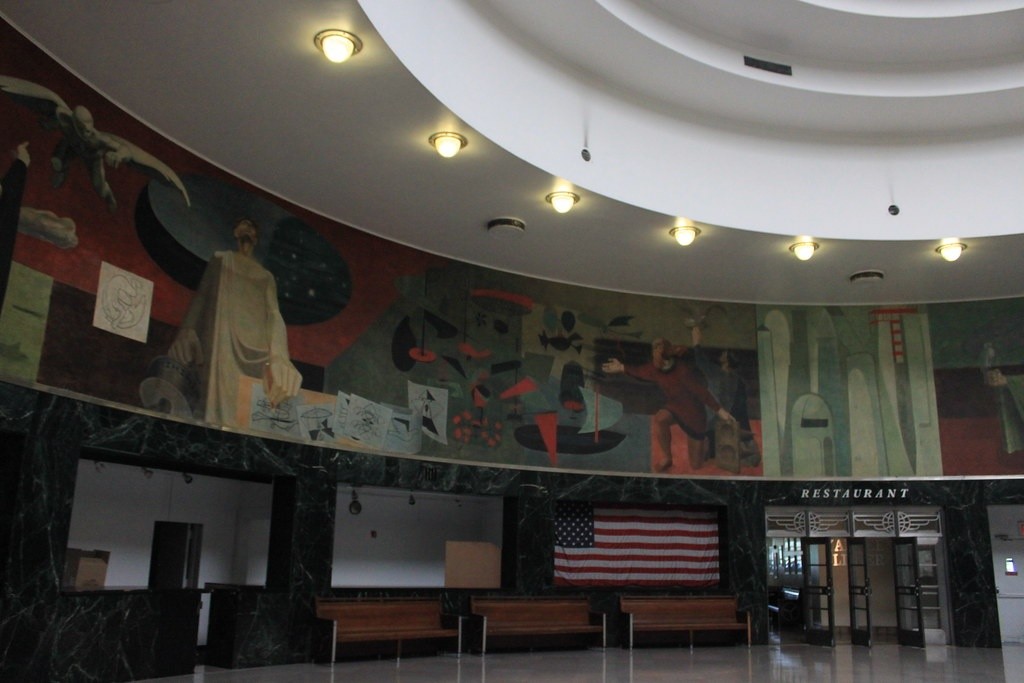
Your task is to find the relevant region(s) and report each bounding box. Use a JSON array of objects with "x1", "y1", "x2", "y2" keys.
[
  {"x1": 618, "y1": 592, "x2": 753, "y2": 651},
  {"x1": 471, "y1": 596, "x2": 606, "y2": 654},
  {"x1": 307, "y1": 599, "x2": 462, "y2": 666}
]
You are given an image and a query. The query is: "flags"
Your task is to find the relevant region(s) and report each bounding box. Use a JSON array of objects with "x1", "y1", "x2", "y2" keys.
[{"x1": 553, "y1": 501, "x2": 719, "y2": 586}]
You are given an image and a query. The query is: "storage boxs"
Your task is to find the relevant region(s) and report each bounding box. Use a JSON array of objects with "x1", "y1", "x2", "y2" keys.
[{"x1": 65, "y1": 548, "x2": 111, "y2": 589}]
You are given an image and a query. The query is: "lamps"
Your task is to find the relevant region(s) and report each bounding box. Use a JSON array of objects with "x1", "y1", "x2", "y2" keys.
[
  {"x1": 181, "y1": 472, "x2": 194, "y2": 485},
  {"x1": 348, "y1": 490, "x2": 362, "y2": 514},
  {"x1": 315, "y1": 29, "x2": 362, "y2": 63},
  {"x1": 409, "y1": 495, "x2": 415, "y2": 505},
  {"x1": 429, "y1": 133, "x2": 467, "y2": 159},
  {"x1": 670, "y1": 226, "x2": 701, "y2": 247},
  {"x1": 789, "y1": 242, "x2": 820, "y2": 261},
  {"x1": 936, "y1": 243, "x2": 967, "y2": 262},
  {"x1": 93, "y1": 458, "x2": 106, "y2": 473},
  {"x1": 139, "y1": 466, "x2": 153, "y2": 478},
  {"x1": 546, "y1": 191, "x2": 580, "y2": 215}
]
